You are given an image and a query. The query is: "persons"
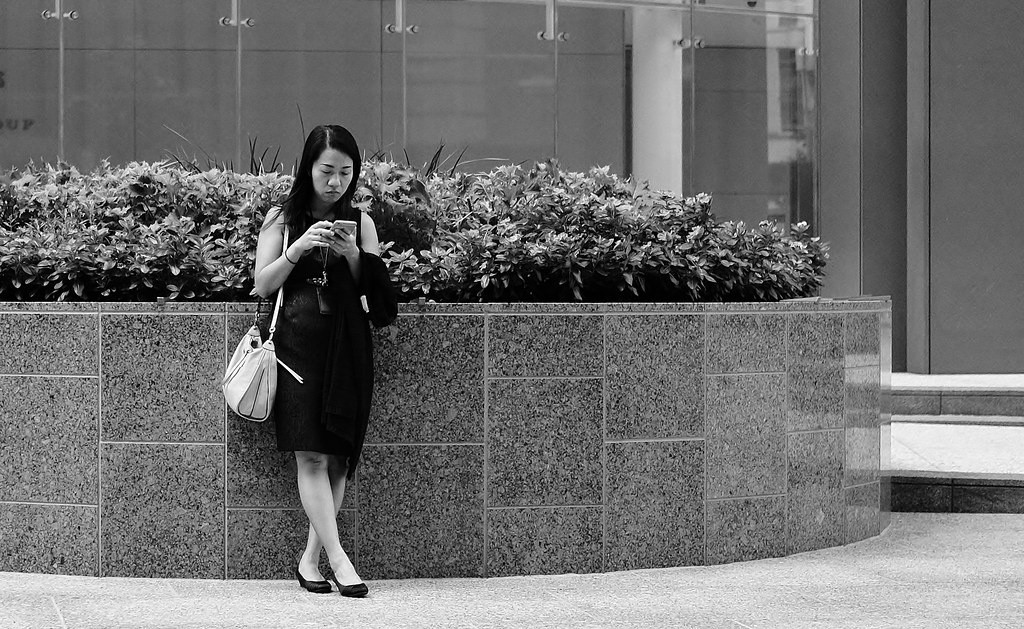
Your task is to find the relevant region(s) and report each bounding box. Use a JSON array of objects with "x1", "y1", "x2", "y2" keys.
[{"x1": 255, "y1": 124, "x2": 380, "y2": 597}]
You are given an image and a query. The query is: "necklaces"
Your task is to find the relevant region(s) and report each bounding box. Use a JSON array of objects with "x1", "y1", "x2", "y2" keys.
[{"x1": 307, "y1": 246, "x2": 329, "y2": 286}]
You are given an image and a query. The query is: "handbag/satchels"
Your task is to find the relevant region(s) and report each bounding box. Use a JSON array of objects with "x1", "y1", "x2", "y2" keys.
[{"x1": 221, "y1": 326, "x2": 277, "y2": 422}]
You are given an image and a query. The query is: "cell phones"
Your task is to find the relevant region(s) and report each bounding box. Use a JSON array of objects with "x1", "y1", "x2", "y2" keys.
[{"x1": 328, "y1": 220, "x2": 357, "y2": 241}]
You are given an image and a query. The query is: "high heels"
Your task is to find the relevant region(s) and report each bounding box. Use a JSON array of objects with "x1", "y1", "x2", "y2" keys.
[
  {"x1": 331, "y1": 570, "x2": 368, "y2": 597},
  {"x1": 295, "y1": 563, "x2": 332, "y2": 593}
]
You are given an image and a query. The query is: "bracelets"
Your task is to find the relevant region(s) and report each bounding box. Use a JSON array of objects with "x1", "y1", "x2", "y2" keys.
[{"x1": 285, "y1": 249, "x2": 300, "y2": 264}]
